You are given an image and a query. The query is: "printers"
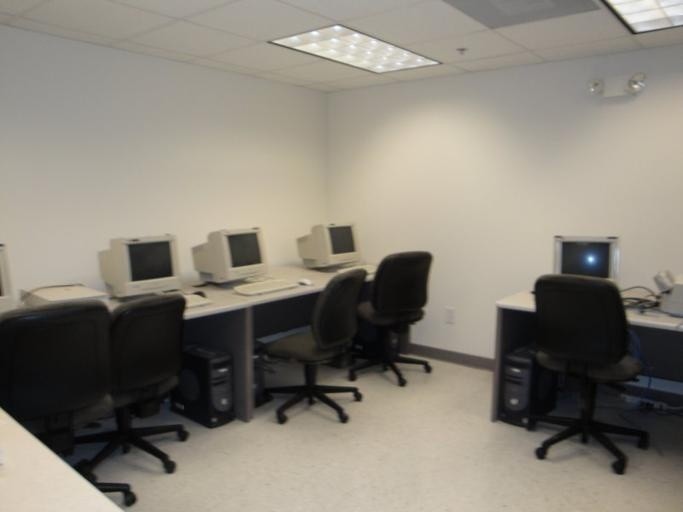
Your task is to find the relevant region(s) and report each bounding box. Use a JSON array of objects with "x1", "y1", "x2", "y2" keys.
[{"x1": 653, "y1": 269, "x2": 683, "y2": 317}]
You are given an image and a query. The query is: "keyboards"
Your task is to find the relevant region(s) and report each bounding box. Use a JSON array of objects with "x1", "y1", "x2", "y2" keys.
[
  {"x1": 184, "y1": 294, "x2": 214, "y2": 308},
  {"x1": 234, "y1": 278, "x2": 298, "y2": 295},
  {"x1": 337, "y1": 263, "x2": 378, "y2": 274}
]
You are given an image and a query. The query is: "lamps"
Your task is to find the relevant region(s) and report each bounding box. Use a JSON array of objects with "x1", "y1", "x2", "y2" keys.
[{"x1": 585, "y1": 71, "x2": 646, "y2": 99}]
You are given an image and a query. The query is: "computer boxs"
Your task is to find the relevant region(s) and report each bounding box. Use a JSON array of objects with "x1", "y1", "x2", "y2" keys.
[
  {"x1": 497, "y1": 335, "x2": 557, "y2": 431},
  {"x1": 170, "y1": 344, "x2": 235, "y2": 428},
  {"x1": 23, "y1": 283, "x2": 109, "y2": 308}
]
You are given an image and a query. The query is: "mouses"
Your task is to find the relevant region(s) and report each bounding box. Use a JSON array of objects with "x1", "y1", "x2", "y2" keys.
[
  {"x1": 298, "y1": 279, "x2": 313, "y2": 287},
  {"x1": 193, "y1": 291, "x2": 206, "y2": 298}
]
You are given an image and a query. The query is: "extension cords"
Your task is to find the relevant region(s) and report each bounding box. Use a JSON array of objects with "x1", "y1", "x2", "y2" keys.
[{"x1": 623, "y1": 393, "x2": 669, "y2": 412}]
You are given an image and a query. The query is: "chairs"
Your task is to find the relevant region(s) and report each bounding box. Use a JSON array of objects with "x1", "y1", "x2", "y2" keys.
[
  {"x1": 263, "y1": 268, "x2": 366, "y2": 424},
  {"x1": 336, "y1": 251, "x2": 432, "y2": 386},
  {"x1": 528, "y1": 275, "x2": 650, "y2": 474},
  {"x1": 0, "y1": 299, "x2": 136, "y2": 506},
  {"x1": 63, "y1": 294, "x2": 189, "y2": 474}
]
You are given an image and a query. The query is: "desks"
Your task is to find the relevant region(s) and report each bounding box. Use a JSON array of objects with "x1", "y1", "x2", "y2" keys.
[
  {"x1": 0, "y1": 408, "x2": 125, "y2": 512},
  {"x1": 107, "y1": 263, "x2": 378, "y2": 422},
  {"x1": 490, "y1": 288, "x2": 683, "y2": 422}
]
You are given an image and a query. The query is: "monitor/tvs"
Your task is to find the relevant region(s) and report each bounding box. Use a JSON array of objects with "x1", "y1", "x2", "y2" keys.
[
  {"x1": 0, "y1": 244, "x2": 23, "y2": 314},
  {"x1": 296, "y1": 223, "x2": 360, "y2": 268},
  {"x1": 191, "y1": 226, "x2": 268, "y2": 283},
  {"x1": 99, "y1": 234, "x2": 182, "y2": 299},
  {"x1": 552, "y1": 235, "x2": 621, "y2": 287}
]
